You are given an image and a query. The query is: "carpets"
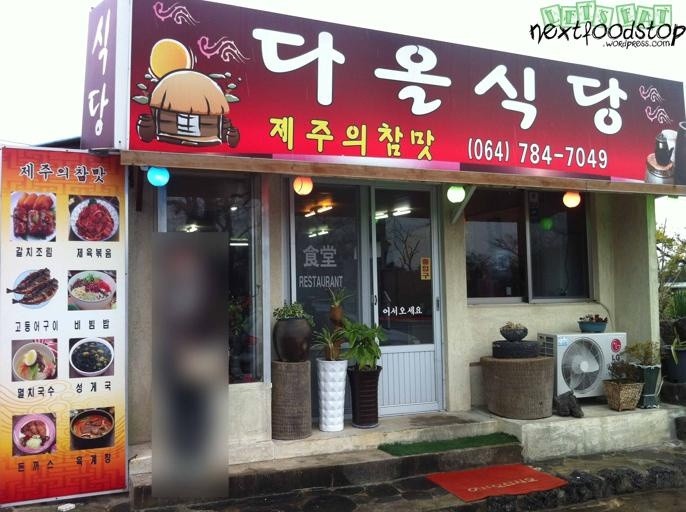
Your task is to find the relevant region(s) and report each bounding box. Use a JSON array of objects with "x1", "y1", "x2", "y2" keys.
[
  {"x1": 425, "y1": 463, "x2": 569, "y2": 502},
  {"x1": 378, "y1": 432, "x2": 521, "y2": 457}
]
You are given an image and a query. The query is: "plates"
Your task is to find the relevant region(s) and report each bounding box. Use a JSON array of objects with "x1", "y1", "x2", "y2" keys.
[
  {"x1": 13, "y1": 194, "x2": 56, "y2": 244},
  {"x1": 11, "y1": 263, "x2": 60, "y2": 305},
  {"x1": 71, "y1": 197, "x2": 118, "y2": 241},
  {"x1": 12, "y1": 413, "x2": 56, "y2": 454}
]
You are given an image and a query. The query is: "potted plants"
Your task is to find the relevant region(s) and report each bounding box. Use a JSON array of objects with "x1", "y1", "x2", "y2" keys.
[
  {"x1": 672, "y1": 290, "x2": 686, "y2": 341},
  {"x1": 308, "y1": 324, "x2": 350, "y2": 432},
  {"x1": 603, "y1": 359, "x2": 646, "y2": 411},
  {"x1": 658, "y1": 288, "x2": 679, "y2": 345},
  {"x1": 577, "y1": 314, "x2": 608, "y2": 333},
  {"x1": 273, "y1": 299, "x2": 316, "y2": 362},
  {"x1": 663, "y1": 325, "x2": 686, "y2": 384},
  {"x1": 343, "y1": 316, "x2": 391, "y2": 429},
  {"x1": 619, "y1": 342, "x2": 668, "y2": 409},
  {"x1": 324, "y1": 286, "x2": 353, "y2": 322},
  {"x1": 499, "y1": 322, "x2": 528, "y2": 341}
]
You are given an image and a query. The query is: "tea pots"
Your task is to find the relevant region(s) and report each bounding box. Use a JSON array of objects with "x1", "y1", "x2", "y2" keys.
[{"x1": 656, "y1": 133, "x2": 675, "y2": 166}]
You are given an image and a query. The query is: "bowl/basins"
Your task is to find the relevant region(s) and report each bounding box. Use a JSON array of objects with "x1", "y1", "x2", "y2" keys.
[
  {"x1": 67, "y1": 272, "x2": 119, "y2": 449},
  {"x1": 13, "y1": 343, "x2": 58, "y2": 383}
]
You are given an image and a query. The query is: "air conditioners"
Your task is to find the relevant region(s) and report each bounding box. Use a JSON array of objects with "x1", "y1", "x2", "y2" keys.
[{"x1": 537, "y1": 333, "x2": 628, "y2": 398}]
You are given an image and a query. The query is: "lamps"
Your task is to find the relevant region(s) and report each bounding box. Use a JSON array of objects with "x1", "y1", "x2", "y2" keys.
[
  {"x1": 146, "y1": 166, "x2": 170, "y2": 187},
  {"x1": 563, "y1": 191, "x2": 580, "y2": 207},
  {"x1": 293, "y1": 177, "x2": 313, "y2": 195},
  {"x1": 447, "y1": 185, "x2": 466, "y2": 204}
]
